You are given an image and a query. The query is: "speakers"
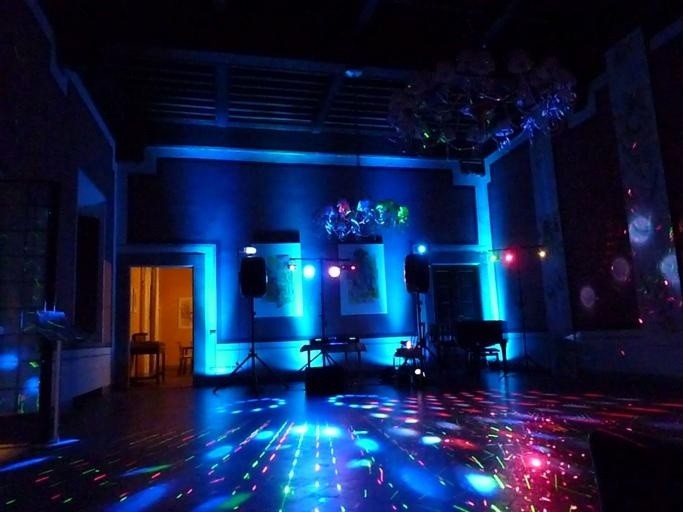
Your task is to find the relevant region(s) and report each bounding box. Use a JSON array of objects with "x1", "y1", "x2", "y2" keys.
[
  {"x1": 239, "y1": 257, "x2": 267, "y2": 296},
  {"x1": 404, "y1": 254, "x2": 430, "y2": 293}
]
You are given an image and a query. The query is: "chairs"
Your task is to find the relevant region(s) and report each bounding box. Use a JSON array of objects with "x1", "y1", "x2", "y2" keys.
[{"x1": 176, "y1": 341, "x2": 193, "y2": 376}]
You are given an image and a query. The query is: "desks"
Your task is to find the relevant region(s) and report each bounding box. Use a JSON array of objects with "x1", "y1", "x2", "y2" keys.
[{"x1": 132, "y1": 340, "x2": 166, "y2": 385}]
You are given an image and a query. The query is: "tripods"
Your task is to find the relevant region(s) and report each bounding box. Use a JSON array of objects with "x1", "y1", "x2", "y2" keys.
[
  {"x1": 397, "y1": 294, "x2": 436, "y2": 367},
  {"x1": 212, "y1": 299, "x2": 288, "y2": 398},
  {"x1": 298, "y1": 260, "x2": 337, "y2": 371}
]
